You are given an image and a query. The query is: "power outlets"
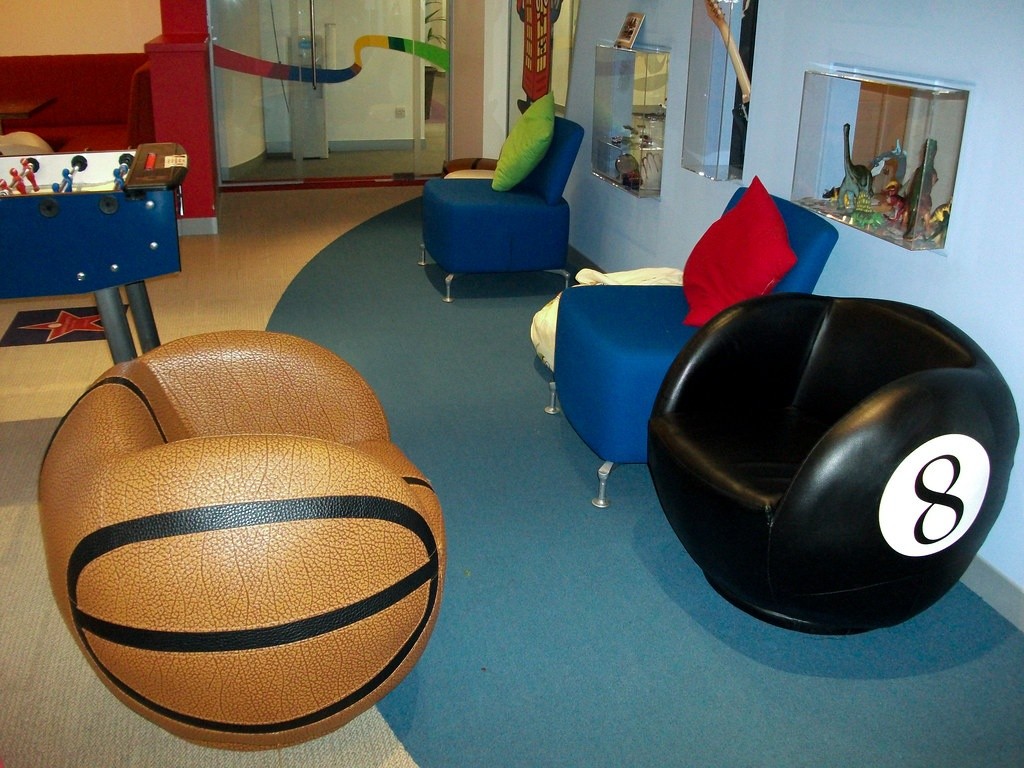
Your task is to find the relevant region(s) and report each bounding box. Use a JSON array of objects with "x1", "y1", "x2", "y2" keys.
[{"x1": 396, "y1": 108, "x2": 405, "y2": 118}]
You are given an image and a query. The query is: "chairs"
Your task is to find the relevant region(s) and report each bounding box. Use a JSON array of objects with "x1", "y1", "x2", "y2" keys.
[
  {"x1": 641, "y1": 293, "x2": 1021, "y2": 635},
  {"x1": 38, "y1": 329, "x2": 445, "y2": 753},
  {"x1": 545, "y1": 187, "x2": 837, "y2": 510},
  {"x1": 419, "y1": 115, "x2": 583, "y2": 303}
]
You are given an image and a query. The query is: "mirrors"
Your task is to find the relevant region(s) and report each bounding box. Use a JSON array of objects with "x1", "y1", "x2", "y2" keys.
[{"x1": 681, "y1": 0, "x2": 760, "y2": 182}]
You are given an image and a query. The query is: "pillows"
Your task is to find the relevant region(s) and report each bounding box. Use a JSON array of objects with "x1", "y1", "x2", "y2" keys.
[
  {"x1": 683, "y1": 179, "x2": 794, "y2": 326},
  {"x1": 492, "y1": 90, "x2": 556, "y2": 190}
]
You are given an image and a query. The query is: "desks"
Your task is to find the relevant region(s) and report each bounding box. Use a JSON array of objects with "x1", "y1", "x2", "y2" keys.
[{"x1": 0, "y1": 96, "x2": 58, "y2": 134}]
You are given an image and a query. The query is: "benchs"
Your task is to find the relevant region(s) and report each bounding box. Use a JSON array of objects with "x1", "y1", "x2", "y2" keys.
[{"x1": 0, "y1": 54, "x2": 149, "y2": 152}]
